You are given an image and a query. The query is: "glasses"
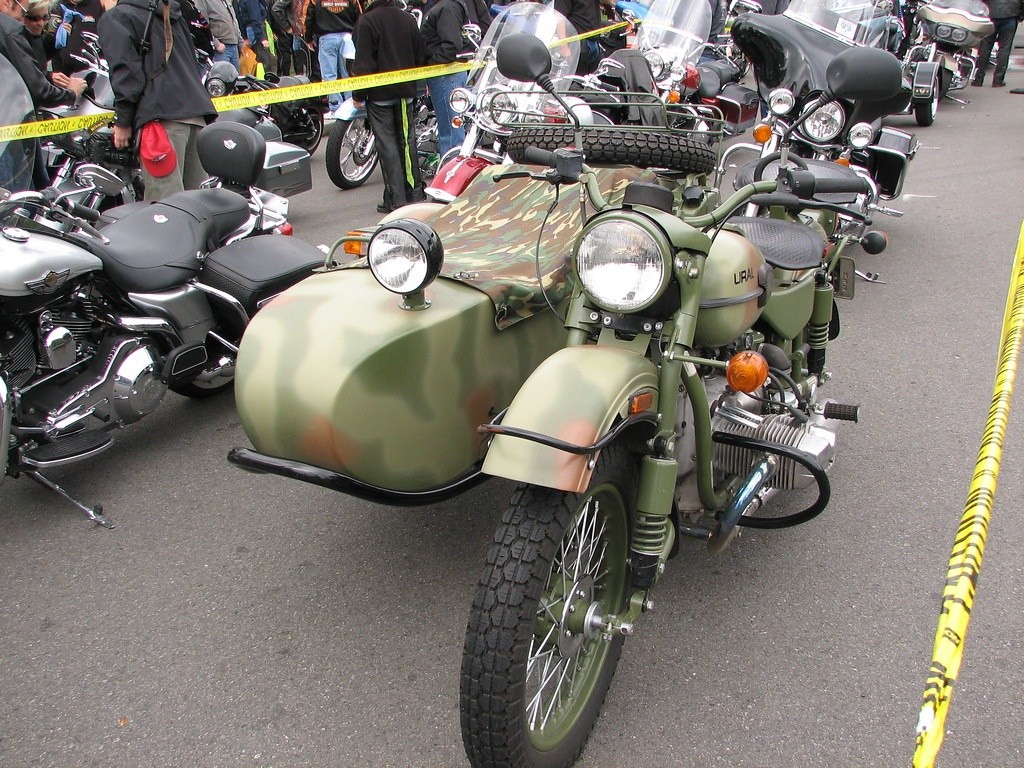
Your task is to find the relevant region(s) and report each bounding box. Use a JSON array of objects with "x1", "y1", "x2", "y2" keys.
[
  {"x1": 15, "y1": 0, "x2": 30, "y2": 18},
  {"x1": 24, "y1": 11, "x2": 52, "y2": 21}
]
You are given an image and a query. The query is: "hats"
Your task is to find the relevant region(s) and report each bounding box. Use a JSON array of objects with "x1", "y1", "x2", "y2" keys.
[{"x1": 139, "y1": 121, "x2": 177, "y2": 178}]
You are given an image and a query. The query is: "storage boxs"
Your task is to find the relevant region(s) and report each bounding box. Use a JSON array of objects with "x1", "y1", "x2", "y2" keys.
[
  {"x1": 201, "y1": 232, "x2": 335, "y2": 331},
  {"x1": 875, "y1": 125, "x2": 921, "y2": 193},
  {"x1": 255, "y1": 141, "x2": 312, "y2": 198},
  {"x1": 721, "y1": 83, "x2": 761, "y2": 133}
]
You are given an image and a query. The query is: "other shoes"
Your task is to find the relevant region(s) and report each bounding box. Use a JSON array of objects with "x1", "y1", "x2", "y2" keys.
[
  {"x1": 992, "y1": 81, "x2": 1006, "y2": 87},
  {"x1": 376, "y1": 204, "x2": 391, "y2": 212},
  {"x1": 324, "y1": 110, "x2": 336, "y2": 119},
  {"x1": 971, "y1": 80, "x2": 982, "y2": 86}
]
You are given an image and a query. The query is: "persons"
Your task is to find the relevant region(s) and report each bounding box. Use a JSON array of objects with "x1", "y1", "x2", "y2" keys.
[
  {"x1": 97, "y1": 0, "x2": 219, "y2": 204},
  {"x1": 178, "y1": 0, "x2": 214, "y2": 63},
  {"x1": 0, "y1": 0, "x2": 106, "y2": 188},
  {"x1": 762, "y1": 0, "x2": 791, "y2": 15},
  {"x1": 302, "y1": 0, "x2": 363, "y2": 120},
  {"x1": 351, "y1": 0, "x2": 427, "y2": 212},
  {"x1": 971, "y1": 0, "x2": 1024, "y2": 86},
  {"x1": 409, "y1": 0, "x2": 492, "y2": 158},
  {"x1": 237, "y1": 0, "x2": 305, "y2": 76},
  {"x1": 556, "y1": 0, "x2": 612, "y2": 77},
  {"x1": 699, "y1": 0, "x2": 726, "y2": 63},
  {"x1": 194, "y1": 0, "x2": 249, "y2": 73}
]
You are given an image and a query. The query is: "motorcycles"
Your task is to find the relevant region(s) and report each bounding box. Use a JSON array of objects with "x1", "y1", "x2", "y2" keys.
[
  {"x1": 224, "y1": 30, "x2": 905, "y2": 768},
  {"x1": 0, "y1": 0, "x2": 996, "y2": 529}
]
